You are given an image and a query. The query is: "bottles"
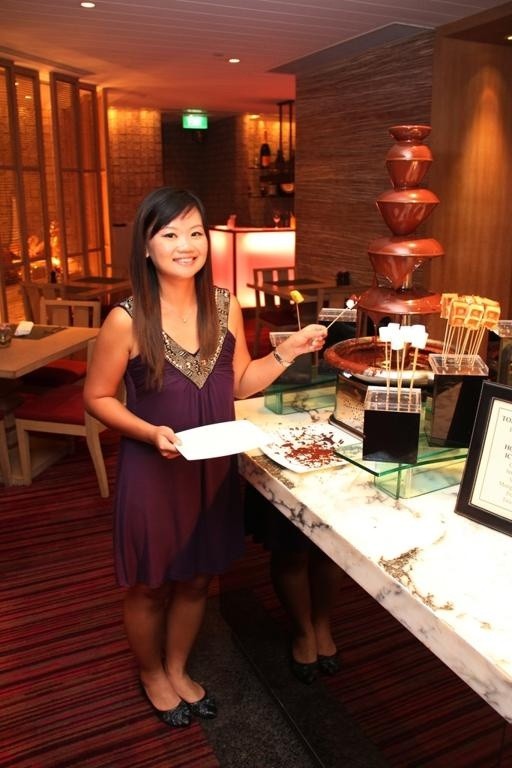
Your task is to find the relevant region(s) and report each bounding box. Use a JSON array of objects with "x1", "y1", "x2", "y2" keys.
[{"x1": 260, "y1": 130, "x2": 271, "y2": 169}]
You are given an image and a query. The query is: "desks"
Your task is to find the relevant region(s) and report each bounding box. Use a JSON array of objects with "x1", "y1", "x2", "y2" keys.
[
  {"x1": 246, "y1": 277, "x2": 353, "y2": 305},
  {"x1": 1, "y1": 324, "x2": 99, "y2": 488},
  {"x1": 53, "y1": 276, "x2": 132, "y2": 300}
]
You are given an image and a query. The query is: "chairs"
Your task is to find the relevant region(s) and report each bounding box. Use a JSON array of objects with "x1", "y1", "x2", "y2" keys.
[
  {"x1": 300, "y1": 285, "x2": 360, "y2": 367},
  {"x1": 20, "y1": 296, "x2": 102, "y2": 393},
  {"x1": 251, "y1": 265, "x2": 302, "y2": 356},
  {"x1": 14, "y1": 335, "x2": 111, "y2": 499},
  {"x1": 18, "y1": 280, "x2": 65, "y2": 323}
]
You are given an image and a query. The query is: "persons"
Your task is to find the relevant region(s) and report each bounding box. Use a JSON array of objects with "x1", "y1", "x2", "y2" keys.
[
  {"x1": 244, "y1": 482, "x2": 342, "y2": 687},
  {"x1": 81, "y1": 186, "x2": 329, "y2": 728}
]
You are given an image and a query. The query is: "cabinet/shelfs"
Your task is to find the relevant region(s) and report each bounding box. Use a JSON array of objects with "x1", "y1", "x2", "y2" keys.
[{"x1": 246, "y1": 163, "x2": 296, "y2": 200}]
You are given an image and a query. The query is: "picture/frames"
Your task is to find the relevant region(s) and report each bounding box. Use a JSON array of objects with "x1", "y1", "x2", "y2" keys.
[{"x1": 453, "y1": 379, "x2": 512, "y2": 537}]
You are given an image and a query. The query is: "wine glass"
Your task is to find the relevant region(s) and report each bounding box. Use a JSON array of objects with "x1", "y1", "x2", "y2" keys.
[{"x1": 272, "y1": 210, "x2": 281, "y2": 228}]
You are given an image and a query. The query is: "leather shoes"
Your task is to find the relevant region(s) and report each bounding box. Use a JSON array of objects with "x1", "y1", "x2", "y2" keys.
[
  {"x1": 291, "y1": 642, "x2": 317, "y2": 684},
  {"x1": 318, "y1": 645, "x2": 345, "y2": 674},
  {"x1": 140, "y1": 681, "x2": 191, "y2": 726},
  {"x1": 182, "y1": 684, "x2": 218, "y2": 719}
]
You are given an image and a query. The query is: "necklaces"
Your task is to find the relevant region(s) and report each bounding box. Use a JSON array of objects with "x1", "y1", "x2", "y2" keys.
[{"x1": 158, "y1": 295, "x2": 200, "y2": 324}]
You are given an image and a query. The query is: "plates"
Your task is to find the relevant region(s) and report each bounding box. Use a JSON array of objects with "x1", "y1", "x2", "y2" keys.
[
  {"x1": 172, "y1": 419, "x2": 274, "y2": 461},
  {"x1": 260, "y1": 422, "x2": 363, "y2": 473}
]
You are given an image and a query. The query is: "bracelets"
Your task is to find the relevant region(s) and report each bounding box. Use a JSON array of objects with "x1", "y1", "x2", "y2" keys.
[{"x1": 268, "y1": 344, "x2": 296, "y2": 371}]
